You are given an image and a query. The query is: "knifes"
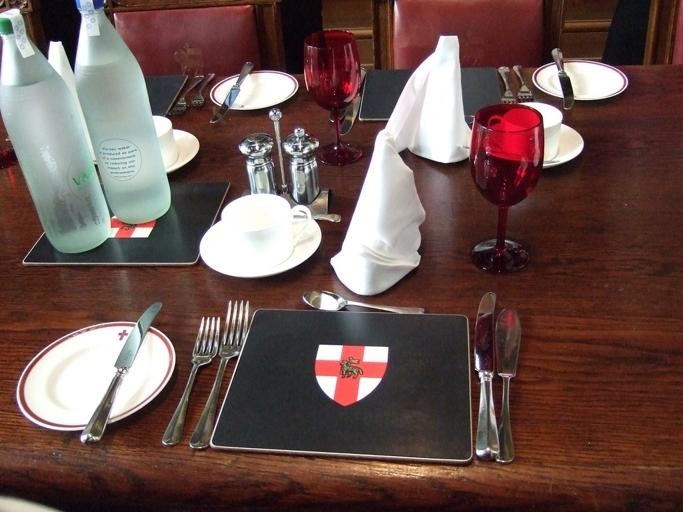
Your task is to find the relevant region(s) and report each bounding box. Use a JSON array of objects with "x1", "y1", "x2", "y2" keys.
[
  {"x1": 552, "y1": 48, "x2": 575, "y2": 111},
  {"x1": 474, "y1": 291, "x2": 500, "y2": 460},
  {"x1": 329, "y1": 70, "x2": 366, "y2": 136},
  {"x1": 494, "y1": 306, "x2": 521, "y2": 464},
  {"x1": 80, "y1": 302, "x2": 162, "y2": 444},
  {"x1": 210, "y1": 62, "x2": 254, "y2": 124}
]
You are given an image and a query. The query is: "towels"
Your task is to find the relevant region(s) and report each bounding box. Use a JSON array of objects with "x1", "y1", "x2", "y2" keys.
[
  {"x1": 47, "y1": 40, "x2": 98, "y2": 164},
  {"x1": 329, "y1": 129, "x2": 427, "y2": 295},
  {"x1": 383, "y1": 35, "x2": 475, "y2": 163}
]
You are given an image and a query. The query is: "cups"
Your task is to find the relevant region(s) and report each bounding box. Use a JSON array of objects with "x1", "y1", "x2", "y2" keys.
[
  {"x1": 152, "y1": 115, "x2": 178, "y2": 169},
  {"x1": 220, "y1": 193, "x2": 311, "y2": 266},
  {"x1": 520, "y1": 102, "x2": 563, "y2": 162}
]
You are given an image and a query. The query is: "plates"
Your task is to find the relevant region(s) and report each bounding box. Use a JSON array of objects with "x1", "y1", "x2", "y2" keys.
[
  {"x1": 198, "y1": 216, "x2": 322, "y2": 279},
  {"x1": 165, "y1": 129, "x2": 200, "y2": 176},
  {"x1": 16, "y1": 322, "x2": 176, "y2": 432},
  {"x1": 531, "y1": 59, "x2": 628, "y2": 101},
  {"x1": 541, "y1": 124, "x2": 584, "y2": 169},
  {"x1": 210, "y1": 70, "x2": 299, "y2": 111}
]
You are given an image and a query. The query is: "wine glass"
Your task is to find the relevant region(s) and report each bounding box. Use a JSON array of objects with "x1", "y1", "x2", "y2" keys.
[
  {"x1": 470, "y1": 104, "x2": 544, "y2": 273},
  {"x1": 304, "y1": 29, "x2": 364, "y2": 166}
]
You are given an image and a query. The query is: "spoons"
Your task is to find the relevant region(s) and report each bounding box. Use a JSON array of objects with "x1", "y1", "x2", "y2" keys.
[{"x1": 300, "y1": 289, "x2": 425, "y2": 316}]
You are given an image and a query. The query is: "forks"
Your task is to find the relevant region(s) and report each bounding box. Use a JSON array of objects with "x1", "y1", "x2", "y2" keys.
[
  {"x1": 161, "y1": 317, "x2": 220, "y2": 450},
  {"x1": 189, "y1": 300, "x2": 249, "y2": 450},
  {"x1": 161, "y1": 73, "x2": 216, "y2": 116},
  {"x1": 498, "y1": 65, "x2": 533, "y2": 104}
]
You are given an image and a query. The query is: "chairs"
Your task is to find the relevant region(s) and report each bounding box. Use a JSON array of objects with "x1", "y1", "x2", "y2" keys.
[
  {"x1": 0, "y1": 0, "x2": 51, "y2": 169},
  {"x1": 372, "y1": 0, "x2": 566, "y2": 70},
  {"x1": 104, "y1": 0, "x2": 288, "y2": 77},
  {"x1": 642, "y1": 0, "x2": 683, "y2": 65}
]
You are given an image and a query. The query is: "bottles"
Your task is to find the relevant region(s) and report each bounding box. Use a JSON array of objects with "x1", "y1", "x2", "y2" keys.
[
  {"x1": 1, "y1": 9, "x2": 111, "y2": 256},
  {"x1": 76, "y1": 0, "x2": 173, "y2": 224},
  {"x1": 239, "y1": 128, "x2": 320, "y2": 206}
]
